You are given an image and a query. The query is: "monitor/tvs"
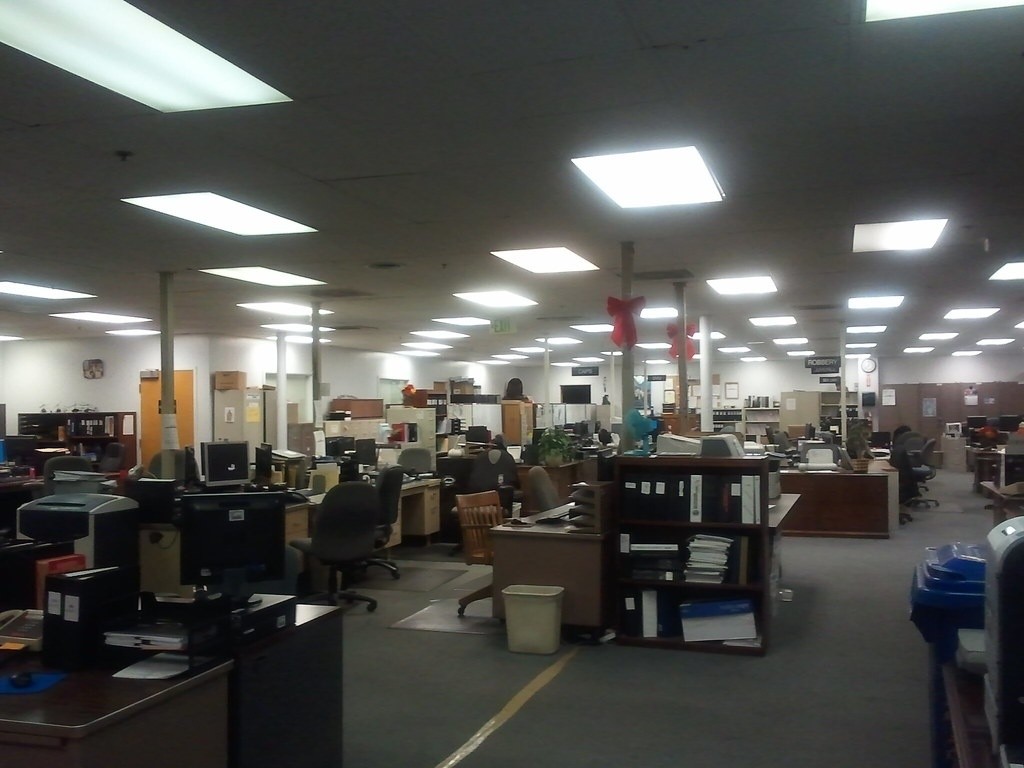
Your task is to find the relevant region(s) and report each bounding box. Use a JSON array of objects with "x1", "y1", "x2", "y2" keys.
[
  {"x1": 967, "y1": 416, "x2": 988, "y2": 429},
  {"x1": 805, "y1": 424, "x2": 816, "y2": 441},
  {"x1": 999, "y1": 416, "x2": 1020, "y2": 432},
  {"x1": 178, "y1": 440, "x2": 287, "y2": 613},
  {"x1": 507, "y1": 446, "x2": 521, "y2": 464},
  {"x1": 596, "y1": 448, "x2": 616, "y2": 482},
  {"x1": 323, "y1": 437, "x2": 383, "y2": 470}
]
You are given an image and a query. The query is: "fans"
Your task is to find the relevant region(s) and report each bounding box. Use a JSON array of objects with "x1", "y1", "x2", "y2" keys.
[{"x1": 621, "y1": 410, "x2": 658, "y2": 454}]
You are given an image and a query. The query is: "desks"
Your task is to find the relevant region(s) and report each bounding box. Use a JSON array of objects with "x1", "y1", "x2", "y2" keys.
[
  {"x1": 488, "y1": 502, "x2": 607, "y2": 630},
  {"x1": 308, "y1": 477, "x2": 442, "y2": 558},
  {"x1": 0, "y1": 601, "x2": 345, "y2": 768},
  {"x1": 778, "y1": 460, "x2": 901, "y2": 539}
]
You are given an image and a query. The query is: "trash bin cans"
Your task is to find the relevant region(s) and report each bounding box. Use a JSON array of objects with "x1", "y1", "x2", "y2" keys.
[
  {"x1": 502, "y1": 584, "x2": 564, "y2": 655},
  {"x1": 912, "y1": 540, "x2": 988, "y2": 768}
]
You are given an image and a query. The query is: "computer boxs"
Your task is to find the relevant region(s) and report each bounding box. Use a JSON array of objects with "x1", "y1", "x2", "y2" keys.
[{"x1": 176, "y1": 593, "x2": 297, "y2": 642}]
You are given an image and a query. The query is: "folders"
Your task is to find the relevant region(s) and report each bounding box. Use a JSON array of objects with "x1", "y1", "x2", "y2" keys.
[{"x1": 42, "y1": 565, "x2": 140, "y2": 672}]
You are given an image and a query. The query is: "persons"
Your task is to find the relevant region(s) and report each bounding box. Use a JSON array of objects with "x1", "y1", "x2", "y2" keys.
[
  {"x1": 1016, "y1": 422, "x2": 1024, "y2": 436},
  {"x1": 502, "y1": 378, "x2": 525, "y2": 400},
  {"x1": 889, "y1": 425, "x2": 925, "y2": 502},
  {"x1": 977, "y1": 420, "x2": 999, "y2": 446},
  {"x1": 468, "y1": 433, "x2": 517, "y2": 494}
]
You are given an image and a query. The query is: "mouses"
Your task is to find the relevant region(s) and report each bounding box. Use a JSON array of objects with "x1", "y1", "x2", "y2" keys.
[{"x1": 511, "y1": 519, "x2": 522, "y2": 524}]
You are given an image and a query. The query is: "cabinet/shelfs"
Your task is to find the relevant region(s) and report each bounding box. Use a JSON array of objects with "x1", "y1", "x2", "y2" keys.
[
  {"x1": 213, "y1": 390, "x2": 265, "y2": 461},
  {"x1": 264, "y1": 390, "x2": 279, "y2": 448},
  {"x1": 713, "y1": 408, "x2": 741, "y2": 433},
  {"x1": 611, "y1": 455, "x2": 772, "y2": 656},
  {"x1": 779, "y1": 392, "x2": 861, "y2": 440},
  {"x1": 16, "y1": 412, "x2": 136, "y2": 469},
  {"x1": 741, "y1": 408, "x2": 781, "y2": 441}
]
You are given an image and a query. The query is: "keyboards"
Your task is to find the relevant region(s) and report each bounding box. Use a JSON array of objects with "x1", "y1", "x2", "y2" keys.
[{"x1": 536, "y1": 510, "x2": 569, "y2": 523}]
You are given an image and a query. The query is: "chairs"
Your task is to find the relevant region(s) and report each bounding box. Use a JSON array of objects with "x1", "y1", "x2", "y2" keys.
[
  {"x1": 761, "y1": 414, "x2": 1024, "y2": 520},
  {"x1": 0, "y1": 418, "x2": 614, "y2": 622}
]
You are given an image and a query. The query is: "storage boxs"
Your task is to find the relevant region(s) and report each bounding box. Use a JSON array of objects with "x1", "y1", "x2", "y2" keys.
[
  {"x1": 434, "y1": 380, "x2": 475, "y2": 396},
  {"x1": 215, "y1": 371, "x2": 248, "y2": 389}
]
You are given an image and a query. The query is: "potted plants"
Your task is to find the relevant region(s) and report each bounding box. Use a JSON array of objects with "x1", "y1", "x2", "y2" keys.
[{"x1": 536, "y1": 428, "x2": 572, "y2": 465}]
